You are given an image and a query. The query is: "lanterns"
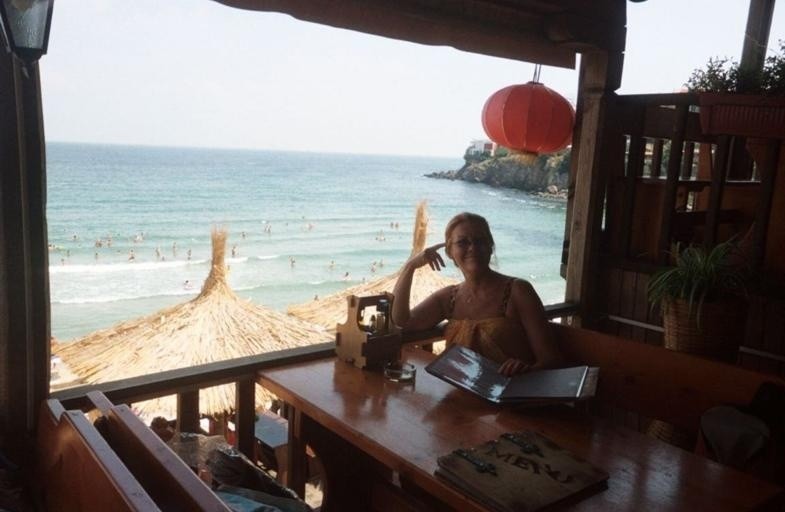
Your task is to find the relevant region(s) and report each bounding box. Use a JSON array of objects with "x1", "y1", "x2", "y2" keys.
[{"x1": 483, "y1": 81, "x2": 574, "y2": 164}]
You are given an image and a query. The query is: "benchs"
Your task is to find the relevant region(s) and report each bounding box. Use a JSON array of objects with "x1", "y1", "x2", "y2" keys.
[
  {"x1": 0, "y1": 399, "x2": 160, "y2": 512},
  {"x1": 549, "y1": 322, "x2": 785, "y2": 480},
  {"x1": 86, "y1": 390, "x2": 312, "y2": 512}
]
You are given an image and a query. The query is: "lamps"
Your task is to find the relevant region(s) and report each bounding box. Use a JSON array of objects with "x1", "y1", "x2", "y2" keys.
[{"x1": 0, "y1": 0, "x2": 54, "y2": 79}]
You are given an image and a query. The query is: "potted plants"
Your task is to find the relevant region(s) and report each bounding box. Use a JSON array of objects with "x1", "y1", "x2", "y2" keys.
[{"x1": 646, "y1": 221, "x2": 769, "y2": 363}]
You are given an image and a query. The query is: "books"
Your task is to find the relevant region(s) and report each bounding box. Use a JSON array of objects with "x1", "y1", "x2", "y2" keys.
[
  {"x1": 424, "y1": 344, "x2": 601, "y2": 410},
  {"x1": 435, "y1": 424, "x2": 609, "y2": 512}
]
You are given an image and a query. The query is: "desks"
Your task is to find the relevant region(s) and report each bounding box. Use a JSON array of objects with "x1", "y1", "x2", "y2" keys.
[{"x1": 255, "y1": 347, "x2": 785, "y2": 512}]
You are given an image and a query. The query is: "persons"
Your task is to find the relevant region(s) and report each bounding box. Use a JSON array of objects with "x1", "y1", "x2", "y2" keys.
[
  {"x1": 226, "y1": 221, "x2": 388, "y2": 301},
  {"x1": 391, "y1": 214, "x2": 556, "y2": 378},
  {"x1": 48, "y1": 231, "x2": 209, "y2": 290}
]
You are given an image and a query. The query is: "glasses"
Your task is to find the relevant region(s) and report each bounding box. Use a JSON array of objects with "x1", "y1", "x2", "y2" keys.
[{"x1": 450, "y1": 238, "x2": 487, "y2": 247}]
